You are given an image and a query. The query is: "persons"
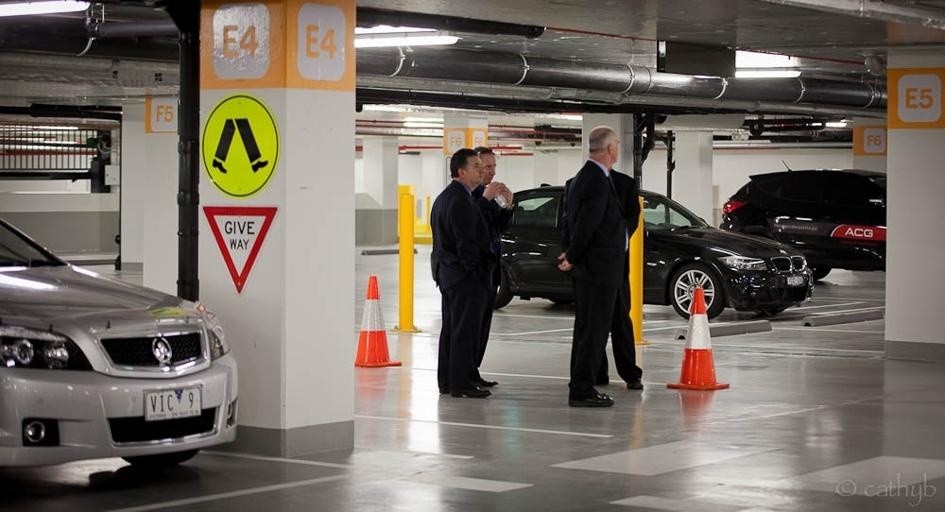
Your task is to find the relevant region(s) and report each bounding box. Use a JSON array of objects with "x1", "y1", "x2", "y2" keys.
[
  {"x1": 429, "y1": 148, "x2": 498, "y2": 399},
  {"x1": 471, "y1": 146, "x2": 514, "y2": 387},
  {"x1": 564, "y1": 165, "x2": 643, "y2": 390},
  {"x1": 555, "y1": 124, "x2": 630, "y2": 407}
]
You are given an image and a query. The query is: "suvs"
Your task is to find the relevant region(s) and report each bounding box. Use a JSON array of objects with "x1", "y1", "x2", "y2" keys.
[{"x1": 719, "y1": 160, "x2": 887, "y2": 281}]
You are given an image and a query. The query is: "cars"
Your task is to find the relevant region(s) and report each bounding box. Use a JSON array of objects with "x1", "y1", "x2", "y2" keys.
[
  {"x1": 495, "y1": 185, "x2": 816, "y2": 321},
  {"x1": 0, "y1": 216, "x2": 239, "y2": 469}
]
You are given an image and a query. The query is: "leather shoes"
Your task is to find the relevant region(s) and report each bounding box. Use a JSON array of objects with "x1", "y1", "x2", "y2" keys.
[
  {"x1": 440, "y1": 378, "x2": 497, "y2": 398},
  {"x1": 569, "y1": 377, "x2": 643, "y2": 407}
]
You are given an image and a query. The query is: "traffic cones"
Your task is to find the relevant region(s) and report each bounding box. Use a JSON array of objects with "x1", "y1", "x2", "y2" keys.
[
  {"x1": 667, "y1": 286, "x2": 730, "y2": 390},
  {"x1": 668, "y1": 390, "x2": 726, "y2": 452},
  {"x1": 355, "y1": 367, "x2": 390, "y2": 445},
  {"x1": 354, "y1": 275, "x2": 402, "y2": 368}
]
